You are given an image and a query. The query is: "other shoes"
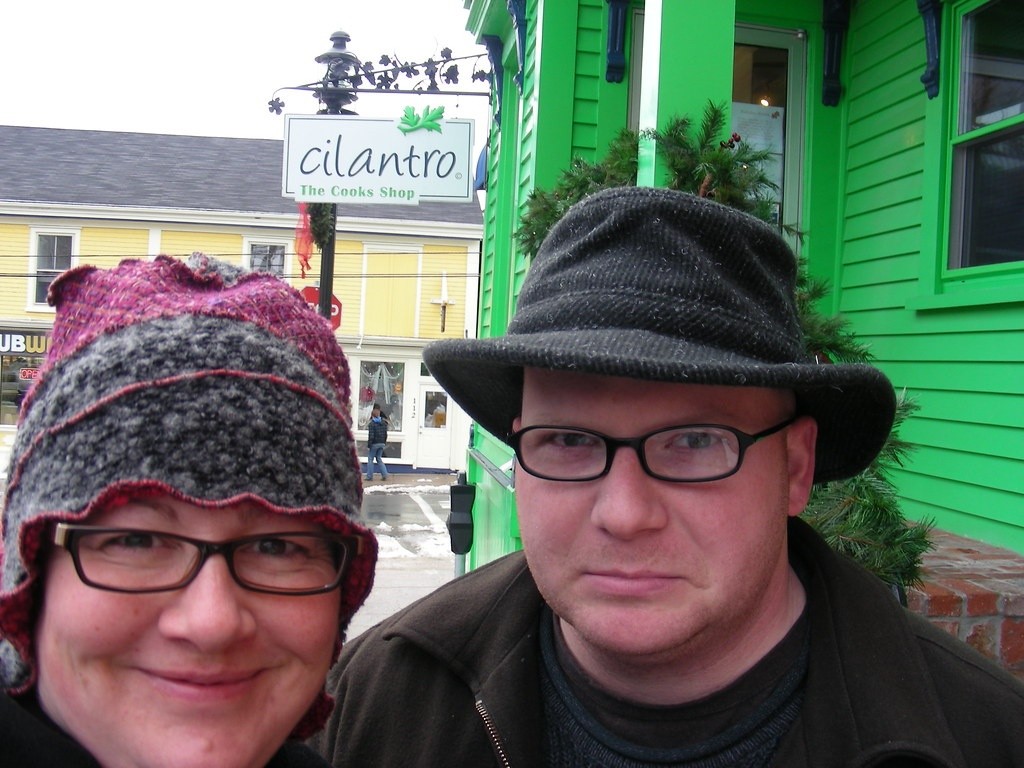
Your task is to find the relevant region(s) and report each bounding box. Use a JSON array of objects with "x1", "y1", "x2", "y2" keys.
[
  {"x1": 381, "y1": 476, "x2": 388, "y2": 481},
  {"x1": 364, "y1": 477, "x2": 372, "y2": 482}
]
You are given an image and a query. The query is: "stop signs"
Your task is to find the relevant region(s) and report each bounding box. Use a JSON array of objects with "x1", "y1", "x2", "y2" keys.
[{"x1": 299, "y1": 286, "x2": 342, "y2": 332}]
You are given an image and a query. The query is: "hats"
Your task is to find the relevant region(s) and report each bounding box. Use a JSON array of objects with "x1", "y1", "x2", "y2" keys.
[
  {"x1": 0, "y1": 250, "x2": 380, "y2": 741},
  {"x1": 423, "y1": 187, "x2": 897, "y2": 485}
]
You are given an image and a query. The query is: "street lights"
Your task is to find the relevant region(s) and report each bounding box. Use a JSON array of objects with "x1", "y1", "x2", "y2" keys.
[{"x1": 313, "y1": 30, "x2": 361, "y2": 323}]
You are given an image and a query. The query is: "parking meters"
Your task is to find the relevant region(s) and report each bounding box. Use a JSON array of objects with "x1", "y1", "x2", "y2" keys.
[{"x1": 447, "y1": 469, "x2": 478, "y2": 578}]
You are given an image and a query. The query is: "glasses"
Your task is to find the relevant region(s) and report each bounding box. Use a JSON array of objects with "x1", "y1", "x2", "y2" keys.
[
  {"x1": 505, "y1": 416, "x2": 796, "y2": 482},
  {"x1": 48, "y1": 519, "x2": 359, "y2": 594}
]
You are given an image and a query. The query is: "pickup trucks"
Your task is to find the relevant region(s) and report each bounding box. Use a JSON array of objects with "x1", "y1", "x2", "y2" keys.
[{"x1": 2, "y1": 371, "x2": 35, "y2": 406}]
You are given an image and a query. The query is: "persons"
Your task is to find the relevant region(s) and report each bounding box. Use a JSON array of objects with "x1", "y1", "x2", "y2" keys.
[
  {"x1": 370, "y1": 404, "x2": 387, "y2": 420},
  {"x1": 295, "y1": 187, "x2": 1024, "y2": 768},
  {"x1": 0, "y1": 253, "x2": 379, "y2": 768},
  {"x1": 364, "y1": 408, "x2": 390, "y2": 481}
]
